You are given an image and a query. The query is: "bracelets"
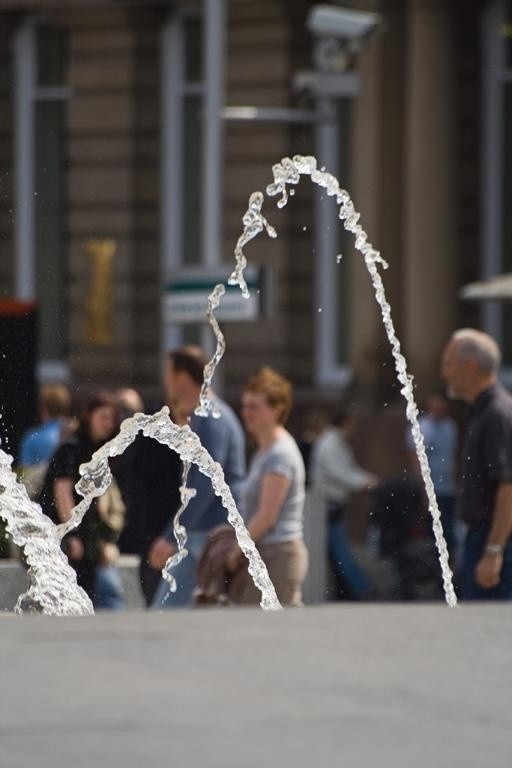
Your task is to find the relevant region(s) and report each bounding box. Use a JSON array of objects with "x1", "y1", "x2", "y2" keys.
[{"x1": 484, "y1": 539, "x2": 505, "y2": 556}]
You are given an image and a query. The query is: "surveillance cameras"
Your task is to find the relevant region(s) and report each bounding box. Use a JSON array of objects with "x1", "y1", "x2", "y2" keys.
[{"x1": 307, "y1": 4, "x2": 380, "y2": 41}]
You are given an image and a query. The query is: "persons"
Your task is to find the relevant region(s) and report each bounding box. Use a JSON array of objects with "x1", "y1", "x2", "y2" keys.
[
  {"x1": 19, "y1": 345, "x2": 307, "y2": 610},
  {"x1": 308, "y1": 377, "x2": 466, "y2": 603},
  {"x1": 435, "y1": 327, "x2": 512, "y2": 601}
]
